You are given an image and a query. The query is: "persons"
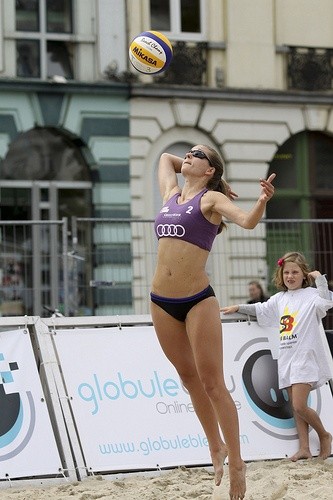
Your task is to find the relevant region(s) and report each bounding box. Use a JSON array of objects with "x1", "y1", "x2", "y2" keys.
[
  {"x1": 244, "y1": 275, "x2": 272, "y2": 306},
  {"x1": 148, "y1": 142, "x2": 278, "y2": 500},
  {"x1": 219, "y1": 250, "x2": 332, "y2": 464}
]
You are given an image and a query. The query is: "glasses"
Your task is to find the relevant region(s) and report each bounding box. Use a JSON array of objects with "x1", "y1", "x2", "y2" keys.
[{"x1": 188, "y1": 150, "x2": 214, "y2": 168}]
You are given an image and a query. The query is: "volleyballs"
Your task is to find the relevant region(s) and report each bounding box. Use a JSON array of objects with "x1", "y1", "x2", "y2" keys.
[{"x1": 129, "y1": 31, "x2": 173, "y2": 75}]
